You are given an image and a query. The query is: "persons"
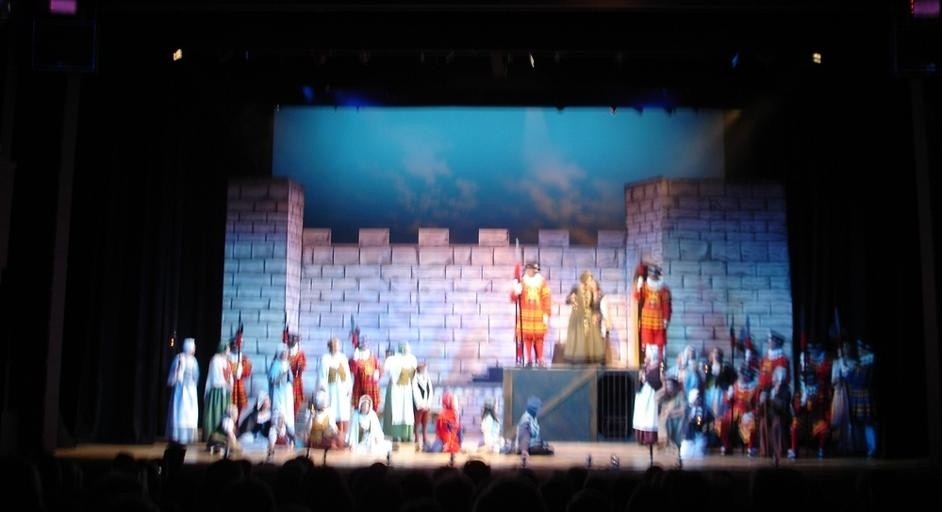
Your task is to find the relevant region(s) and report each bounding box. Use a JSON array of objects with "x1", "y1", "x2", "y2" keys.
[
  {"x1": 511, "y1": 259, "x2": 557, "y2": 366},
  {"x1": 169, "y1": 336, "x2": 465, "y2": 455},
  {"x1": 636, "y1": 258, "x2": 672, "y2": 365},
  {"x1": 566, "y1": 270, "x2": 607, "y2": 362},
  {"x1": 630, "y1": 327, "x2": 879, "y2": 461},
  {"x1": 481, "y1": 400, "x2": 514, "y2": 451},
  {"x1": 515, "y1": 395, "x2": 555, "y2": 456}
]
